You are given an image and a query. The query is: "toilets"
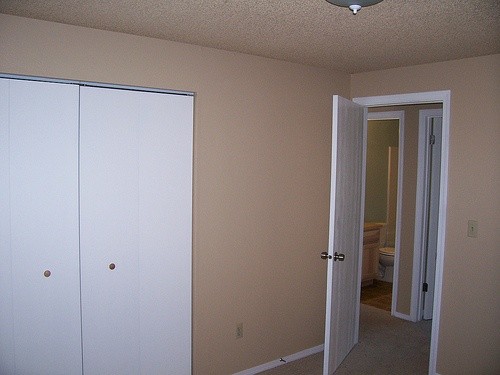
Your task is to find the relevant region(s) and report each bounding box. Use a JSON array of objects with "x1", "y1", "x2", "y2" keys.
[{"x1": 377, "y1": 247, "x2": 395, "y2": 281}]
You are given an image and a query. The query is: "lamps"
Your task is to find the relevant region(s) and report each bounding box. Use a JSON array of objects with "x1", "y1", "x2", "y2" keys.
[{"x1": 326, "y1": 0, "x2": 384, "y2": 15}]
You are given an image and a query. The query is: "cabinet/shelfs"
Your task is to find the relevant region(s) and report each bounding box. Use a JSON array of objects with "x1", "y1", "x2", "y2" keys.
[
  {"x1": 361, "y1": 229, "x2": 380, "y2": 281},
  {"x1": 0, "y1": 72, "x2": 192, "y2": 375}
]
